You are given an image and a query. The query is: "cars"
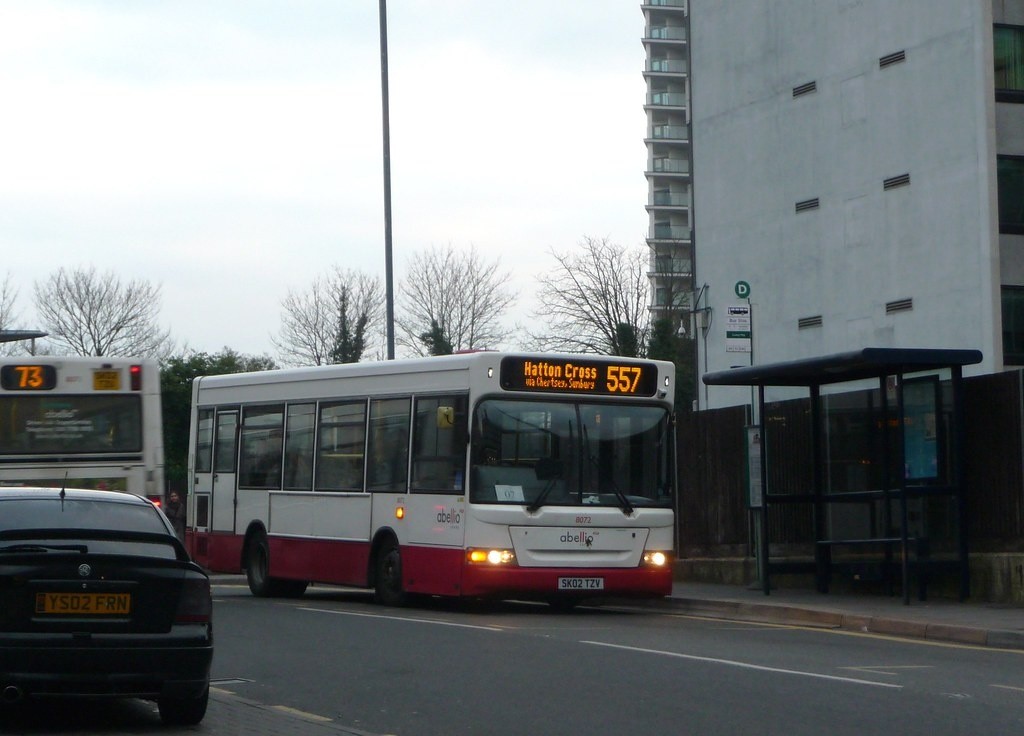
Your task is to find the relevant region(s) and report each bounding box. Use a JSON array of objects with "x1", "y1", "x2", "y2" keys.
[{"x1": 0, "y1": 486, "x2": 213, "y2": 727}]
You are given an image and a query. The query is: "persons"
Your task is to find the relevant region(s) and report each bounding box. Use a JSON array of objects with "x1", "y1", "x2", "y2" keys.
[{"x1": 165, "y1": 492, "x2": 186, "y2": 544}]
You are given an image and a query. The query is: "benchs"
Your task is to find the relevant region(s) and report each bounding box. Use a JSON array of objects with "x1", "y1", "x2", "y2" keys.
[{"x1": 817, "y1": 536, "x2": 920, "y2": 547}]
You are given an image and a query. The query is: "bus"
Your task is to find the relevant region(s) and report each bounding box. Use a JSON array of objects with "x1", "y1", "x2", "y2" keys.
[
  {"x1": 0, "y1": 356, "x2": 167, "y2": 512},
  {"x1": 184, "y1": 350, "x2": 678, "y2": 606}
]
